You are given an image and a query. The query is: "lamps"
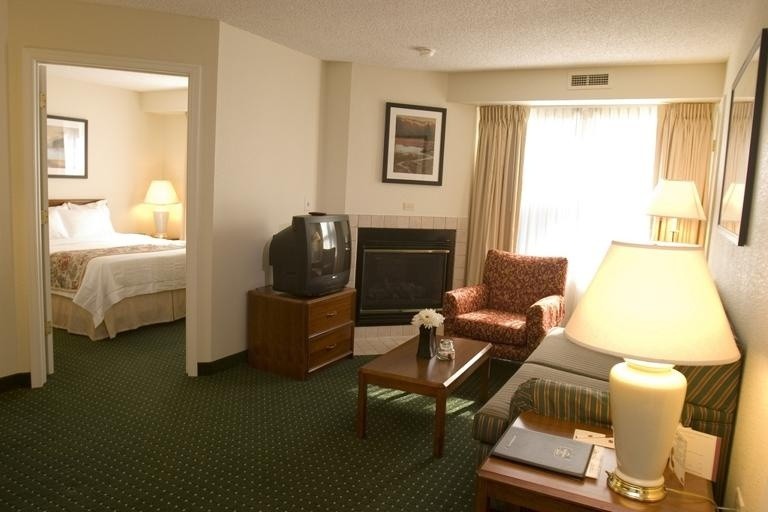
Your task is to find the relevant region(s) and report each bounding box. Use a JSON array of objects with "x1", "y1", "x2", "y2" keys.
[
  {"x1": 564, "y1": 240, "x2": 742, "y2": 504},
  {"x1": 142, "y1": 180, "x2": 179, "y2": 238},
  {"x1": 645, "y1": 178, "x2": 708, "y2": 244}
]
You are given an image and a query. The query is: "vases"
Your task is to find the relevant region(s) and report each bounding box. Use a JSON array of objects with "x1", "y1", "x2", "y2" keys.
[{"x1": 416, "y1": 324, "x2": 437, "y2": 359}]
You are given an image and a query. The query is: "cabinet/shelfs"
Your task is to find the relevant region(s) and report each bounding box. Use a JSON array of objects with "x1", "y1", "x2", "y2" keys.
[{"x1": 245, "y1": 285, "x2": 357, "y2": 380}]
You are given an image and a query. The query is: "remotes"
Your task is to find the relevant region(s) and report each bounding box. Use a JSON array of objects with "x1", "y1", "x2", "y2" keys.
[{"x1": 308, "y1": 212, "x2": 326, "y2": 216}]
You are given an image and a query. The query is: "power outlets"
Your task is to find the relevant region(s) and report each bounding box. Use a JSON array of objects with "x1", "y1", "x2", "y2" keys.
[{"x1": 732, "y1": 487, "x2": 743, "y2": 512}]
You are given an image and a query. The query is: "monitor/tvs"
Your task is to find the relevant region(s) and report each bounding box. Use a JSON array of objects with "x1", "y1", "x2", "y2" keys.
[{"x1": 272, "y1": 214, "x2": 351, "y2": 298}]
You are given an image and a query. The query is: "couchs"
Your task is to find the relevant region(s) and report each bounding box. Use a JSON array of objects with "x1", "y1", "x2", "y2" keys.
[
  {"x1": 477, "y1": 312, "x2": 745, "y2": 511},
  {"x1": 442, "y1": 248, "x2": 568, "y2": 355}
]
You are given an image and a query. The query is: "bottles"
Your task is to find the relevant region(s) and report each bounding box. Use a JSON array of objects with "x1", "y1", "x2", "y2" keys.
[{"x1": 435, "y1": 338, "x2": 456, "y2": 360}]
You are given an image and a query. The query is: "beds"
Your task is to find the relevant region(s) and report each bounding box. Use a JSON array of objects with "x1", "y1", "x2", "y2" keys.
[{"x1": 47, "y1": 198, "x2": 187, "y2": 342}]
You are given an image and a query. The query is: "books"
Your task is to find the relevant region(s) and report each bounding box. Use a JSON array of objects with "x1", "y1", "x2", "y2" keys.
[
  {"x1": 489, "y1": 426, "x2": 597, "y2": 481},
  {"x1": 673, "y1": 424, "x2": 721, "y2": 482}
]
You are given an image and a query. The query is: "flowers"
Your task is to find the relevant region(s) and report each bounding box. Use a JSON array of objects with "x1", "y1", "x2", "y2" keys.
[{"x1": 409, "y1": 308, "x2": 444, "y2": 329}]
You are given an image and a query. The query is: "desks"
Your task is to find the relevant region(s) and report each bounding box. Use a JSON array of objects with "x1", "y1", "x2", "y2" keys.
[{"x1": 473, "y1": 413, "x2": 716, "y2": 512}]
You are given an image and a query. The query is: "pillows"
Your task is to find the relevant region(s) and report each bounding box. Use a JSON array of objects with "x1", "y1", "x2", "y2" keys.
[
  {"x1": 65, "y1": 199, "x2": 116, "y2": 237},
  {"x1": 48, "y1": 202, "x2": 66, "y2": 237}
]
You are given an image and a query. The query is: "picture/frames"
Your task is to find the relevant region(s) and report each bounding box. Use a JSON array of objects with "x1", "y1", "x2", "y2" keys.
[
  {"x1": 381, "y1": 103, "x2": 448, "y2": 185},
  {"x1": 715, "y1": 27, "x2": 765, "y2": 246},
  {"x1": 46, "y1": 114, "x2": 88, "y2": 178}
]
